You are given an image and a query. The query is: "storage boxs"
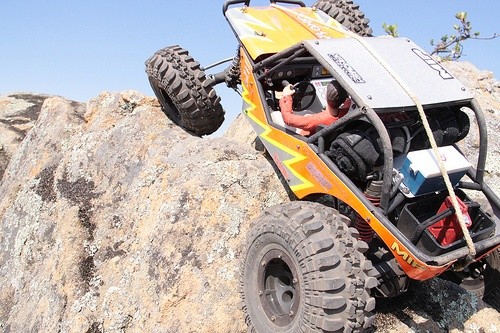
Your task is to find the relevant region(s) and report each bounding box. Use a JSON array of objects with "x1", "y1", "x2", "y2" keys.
[
  {"x1": 397, "y1": 188, "x2": 496, "y2": 256},
  {"x1": 400, "y1": 145, "x2": 472, "y2": 196}
]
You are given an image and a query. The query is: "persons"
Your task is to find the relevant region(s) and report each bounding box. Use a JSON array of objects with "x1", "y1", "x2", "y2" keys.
[{"x1": 271, "y1": 80, "x2": 353, "y2": 139}]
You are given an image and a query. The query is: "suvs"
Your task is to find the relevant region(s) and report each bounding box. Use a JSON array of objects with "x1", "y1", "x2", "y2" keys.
[{"x1": 143, "y1": 1, "x2": 500, "y2": 332}]
